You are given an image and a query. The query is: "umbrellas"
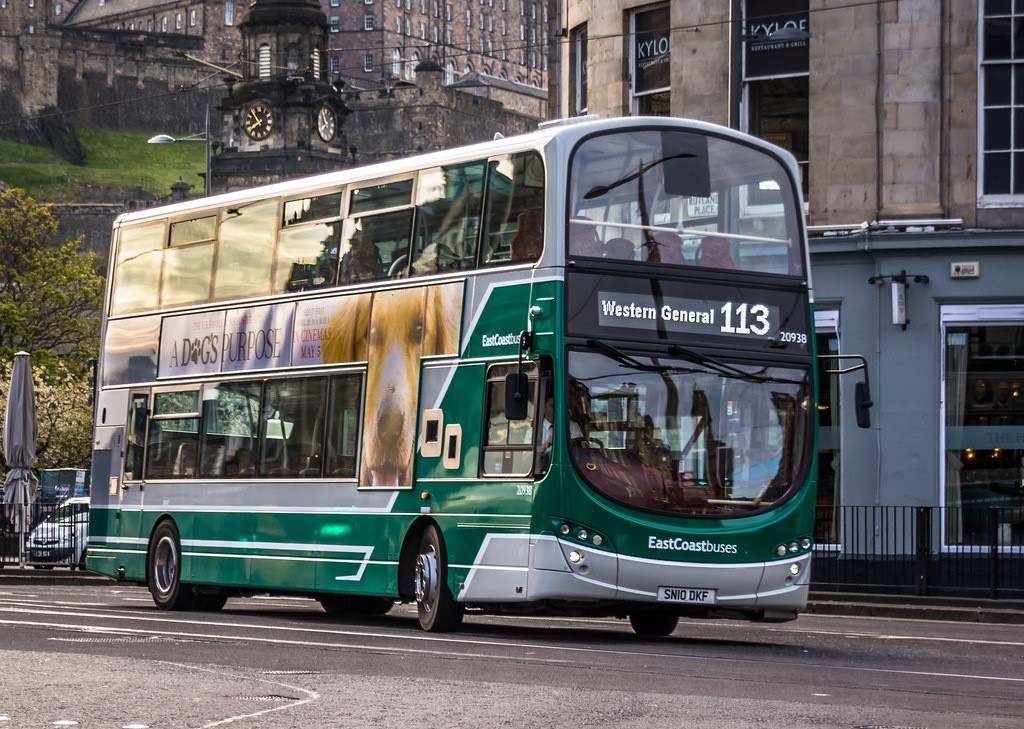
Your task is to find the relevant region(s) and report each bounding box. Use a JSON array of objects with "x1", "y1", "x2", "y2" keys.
[{"x1": 3, "y1": 351, "x2": 39, "y2": 567}]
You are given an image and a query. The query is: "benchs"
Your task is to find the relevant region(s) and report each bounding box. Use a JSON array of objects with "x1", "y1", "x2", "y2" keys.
[{"x1": 239, "y1": 467, "x2": 355, "y2": 477}]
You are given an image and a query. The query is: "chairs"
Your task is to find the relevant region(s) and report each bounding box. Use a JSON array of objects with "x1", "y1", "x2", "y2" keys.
[{"x1": 343, "y1": 211, "x2": 737, "y2": 284}]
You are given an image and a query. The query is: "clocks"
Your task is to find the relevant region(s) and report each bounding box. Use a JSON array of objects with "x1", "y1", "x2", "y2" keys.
[
  {"x1": 316, "y1": 105, "x2": 337, "y2": 142},
  {"x1": 244, "y1": 104, "x2": 274, "y2": 141}
]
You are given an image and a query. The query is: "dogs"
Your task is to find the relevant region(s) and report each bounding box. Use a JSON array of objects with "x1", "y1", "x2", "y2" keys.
[{"x1": 321, "y1": 285, "x2": 461, "y2": 488}]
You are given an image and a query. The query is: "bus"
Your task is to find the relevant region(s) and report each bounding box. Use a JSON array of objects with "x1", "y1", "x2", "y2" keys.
[{"x1": 80, "y1": 110, "x2": 875, "y2": 644}]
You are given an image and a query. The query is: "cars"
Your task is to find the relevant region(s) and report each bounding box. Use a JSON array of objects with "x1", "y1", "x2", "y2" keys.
[{"x1": 21, "y1": 496, "x2": 92, "y2": 572}]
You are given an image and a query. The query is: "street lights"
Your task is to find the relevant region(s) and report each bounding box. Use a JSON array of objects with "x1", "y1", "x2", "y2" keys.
[{"x1": 147, "y1": 101, "x2": 213, "y2": 199}]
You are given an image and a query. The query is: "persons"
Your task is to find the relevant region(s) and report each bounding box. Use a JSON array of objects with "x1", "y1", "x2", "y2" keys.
[{"x1": 522, "y1": 393, "x2": 590, "y2": 467}]
